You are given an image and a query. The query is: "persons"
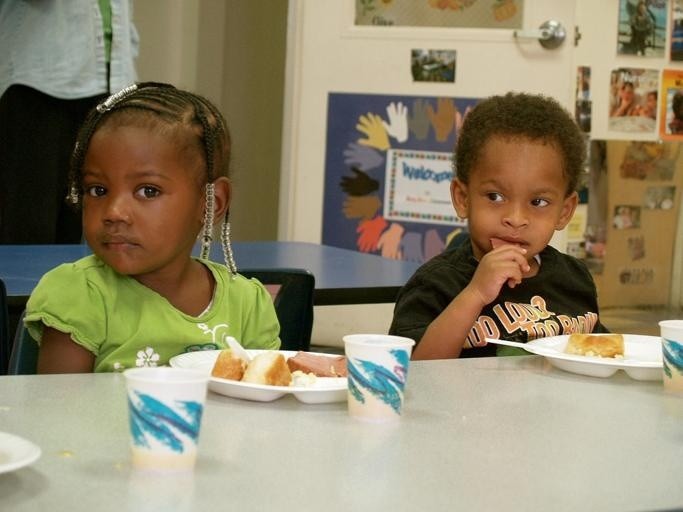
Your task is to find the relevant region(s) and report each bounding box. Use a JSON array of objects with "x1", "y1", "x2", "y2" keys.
[
  {"x1": 630, "y1": 3, "x2": 653, "y2": 56},
  {"x1": 21, "y1": 83, "x2": 283, "y2": 374},
  {"x1": 387, "y1": 92, "x2": 612, "y2": 362},
  {"x1": 610, "y1": 81, "x2": 639, "y2": 118},
  {"x1": 0, "y1": 1, "x2": 138, "y2": 247},
  {"x1": 667, "y1": 92, "x2": 683, "y2": 135},
  {"x1": 640, "y1": 90, "x2": 658, "y2": 117}
]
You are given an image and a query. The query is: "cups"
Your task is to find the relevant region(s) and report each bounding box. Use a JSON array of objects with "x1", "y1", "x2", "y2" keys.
[
  {"x1": 658, "y1": 320, "x2": 683, "y2": 394},
  {"x1": 344, "y1": 335, "x2": 417, "y2": 422},
  {"x1": 122, "y1": 368, "x2": 210, "y2": 471}
]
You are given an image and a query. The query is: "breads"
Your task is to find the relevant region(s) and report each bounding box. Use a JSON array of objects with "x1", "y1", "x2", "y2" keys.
[
  {"x1": 208, "y1": 349, "x2": 247, "y2": 380},
  {"x1": 242, "y1": 348, "x2": 288, "y2": 387},
  {"x1": 569, "y1": 332, "x2": 628, "y2": 359}
]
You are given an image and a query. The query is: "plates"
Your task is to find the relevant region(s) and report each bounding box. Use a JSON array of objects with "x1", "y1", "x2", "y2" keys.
[
  {"x1": 0, "y1": 431, "x2": 42, "y2": 474},
  {"x1": 169, "y1": 349, "x2": 349, "y2": 403},
  {"x1": 521, "y1": 333, "x2": 665, "y2": 381}
]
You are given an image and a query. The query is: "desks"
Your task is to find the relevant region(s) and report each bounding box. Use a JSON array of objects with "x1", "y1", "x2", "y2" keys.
[
  {"x1": 1, "y1": 240, "x2": 431, "y2": 310},
  {"x1": 0, "y1": 354, "x2": 683, "y2": 512}
]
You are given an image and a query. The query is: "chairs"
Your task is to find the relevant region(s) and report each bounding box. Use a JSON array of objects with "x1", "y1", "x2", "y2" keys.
[{"x1": 9, "y1": 263, "x2": 315, "y2": 376}]
